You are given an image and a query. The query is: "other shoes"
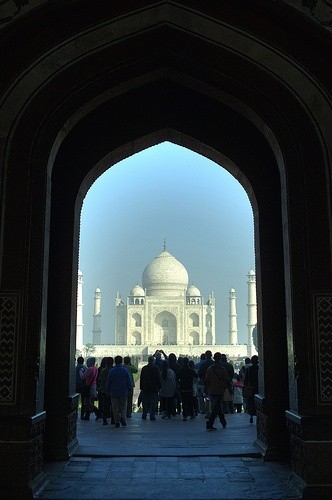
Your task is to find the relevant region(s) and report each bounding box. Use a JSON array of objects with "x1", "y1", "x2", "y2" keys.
[
  {"x1": 127, "y1": 416, "x2": 131, "y2": 418},
  {"x1": 250, "y1": 418, "x2": 253, "y2": 423},
  {"x1": 121, "y1": 424, "x2": 125, "y2": 427},
  {"x1": 81, "y1": 417, "x2": 84, "y2": 419},
  {"x1": 190, "y1": 417, "x2": 195, "y2": 419},
  {"x1": 115, "y1": 424, "x2": 119, "y2": 426},
  {"x1": 224, "y1": 422, "x2": 227, "y2": 429},
  {"x1": 204, "y1": 416, "x2": 209, "y2": 418},
  {"x1": 103, "y1": 422, "x2": 108, "y2": 424},
  {"x1": 111, "y1": 423, "x2": 114, "y2": 424},
  {"x1": 206, "y1": 427, "x2": 217, "y2": 430},
  {"x1": 95, "y1": 418, "x2": 99, "y2": 420},
  {"x1": 85, "y1": 416, "x2": 90, "y2": 421},
  {"x1": 183, "y1": 419, "x2": 187, "y2": 421}
]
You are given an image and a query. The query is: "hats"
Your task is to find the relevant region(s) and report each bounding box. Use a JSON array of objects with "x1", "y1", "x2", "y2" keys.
[{"x1": 88, "y1": 358, "x2": 95, "y2": 365}]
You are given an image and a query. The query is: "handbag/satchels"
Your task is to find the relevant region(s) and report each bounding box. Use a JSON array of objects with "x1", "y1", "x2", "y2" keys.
[
  {"x1": 246, "y1": 387, "x2": 253, "y2": 396},
  {"x1": 83, "y1": 386, "x2": 91, "y2": 397},
  {"x1": 220, "y1": 380, "x2": 226, "y2": 387}
]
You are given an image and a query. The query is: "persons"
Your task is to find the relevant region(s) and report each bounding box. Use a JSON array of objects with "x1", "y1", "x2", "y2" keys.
[
  {"x1": 141, "y1": 349, "x2": 259, "y2": 421},
  {"x1": 203, "y1": 354, "x2": 229, "y2": 430},
  {"x1": 76, "y1": 356, "x2": 138, "y2": 428}
]
